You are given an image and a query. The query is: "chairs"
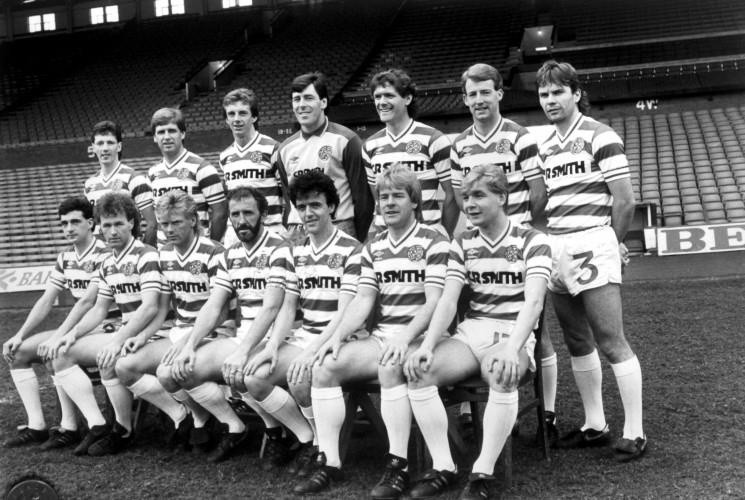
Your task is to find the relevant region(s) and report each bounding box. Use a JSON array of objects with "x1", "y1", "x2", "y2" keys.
[{"x1": 0, "y1": 0, "x2": 745, "y2": 480}]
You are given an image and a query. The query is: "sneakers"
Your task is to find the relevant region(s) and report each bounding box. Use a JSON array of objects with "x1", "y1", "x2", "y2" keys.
[
  {"x1": 259, "y1": 426, "x2": 344, "y2": 496},
  {"x1": 2, "y1": 420, "x2": 135, "y2": 456},
  {"x1": 370, "y1": 452, "x2": 497, "y2": 500},
  {"x1": 534, "y1": 410, "x2": 647, "y2": 461},
  {"x1": 145, "y1": 396, "x2": 255, "y2": 462},
  {"x1": 457, "y1": 412, "x2": 476, "y2": 444}
]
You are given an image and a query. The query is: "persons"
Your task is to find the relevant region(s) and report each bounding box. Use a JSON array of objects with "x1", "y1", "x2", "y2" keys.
[
  {"x1": 536, "y1": 59, "x2": 647, "y2": 464},
  {"x1": 4, "y1": 62, "x2": 551, "y2": 500}
]
